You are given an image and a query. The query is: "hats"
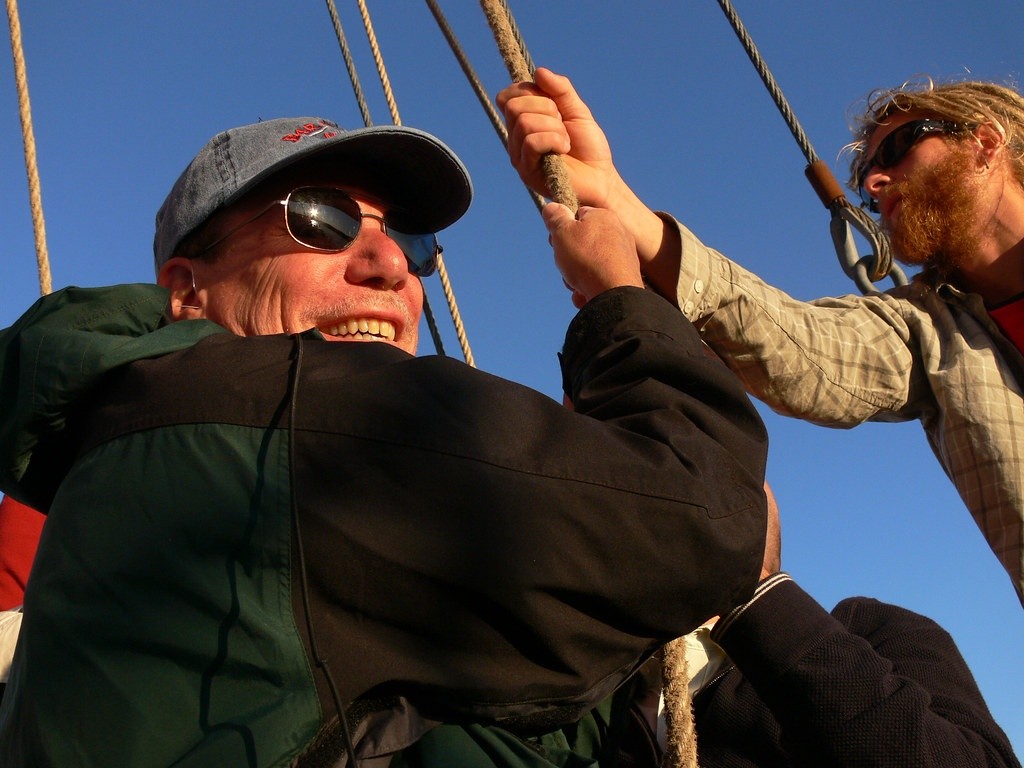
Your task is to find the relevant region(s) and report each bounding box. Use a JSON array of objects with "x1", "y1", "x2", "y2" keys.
[{"x1": 153, "y1": 117, "x2": 474, "y2": 280}]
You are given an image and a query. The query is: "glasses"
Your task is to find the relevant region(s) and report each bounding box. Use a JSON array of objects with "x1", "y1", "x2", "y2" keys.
[
  {"x1": 191, "y1": 184, "x2": 442, "y2": 278},
  {"x1": 856, "y1": 119, "x2": 979, "y2": 214}
]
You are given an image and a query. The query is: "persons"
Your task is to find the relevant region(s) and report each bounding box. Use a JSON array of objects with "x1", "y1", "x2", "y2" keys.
[
  {"x1": 496, "y1": 66, "x2": 1024, "y2": 609},
  {"x1": 564, "y1": 393, "x2": 1022, "y2": 768},
  {"x1": 0, "y1": 492, "x2": 48, "y2": 702},
  {"x1": 0, "y1": 116, "x2": 769, "y2": 768}
]
